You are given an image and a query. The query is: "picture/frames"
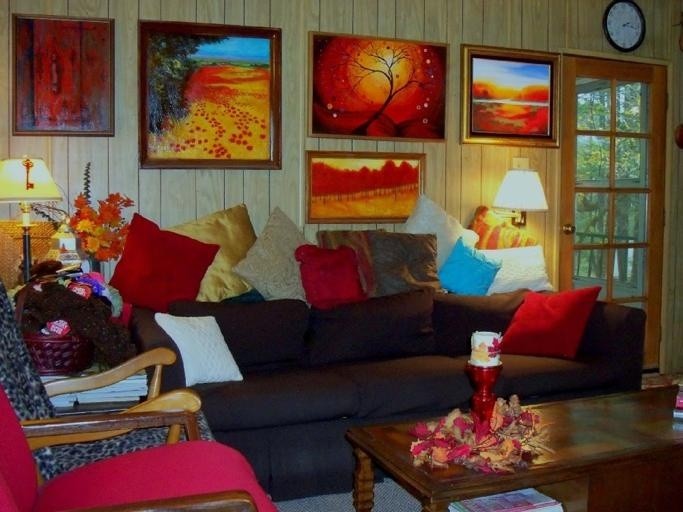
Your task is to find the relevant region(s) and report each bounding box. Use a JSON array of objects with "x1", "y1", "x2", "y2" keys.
[
  {"x1": 459, "y1": 43, "x2": 563, "y2": 151},
  {"x1": 135, "y1": 18, "x2": 283, "y2": 173},
  {"x1": 303, "y1": 148, "x2": 425, "y2": 225},
  {"x1": 10, "y1": 11, "x2": 117, "y2": 137},
  {"x1": 302, "y1": 28, "x2": 449, "y2": 145}
]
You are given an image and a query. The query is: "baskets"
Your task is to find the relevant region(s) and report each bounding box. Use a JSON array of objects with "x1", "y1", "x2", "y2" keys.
[{"x1": 15, "y1": 281, "x2": 93, "y2": 375}]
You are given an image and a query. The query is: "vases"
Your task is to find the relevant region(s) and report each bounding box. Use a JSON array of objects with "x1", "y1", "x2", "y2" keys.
[{"x1": 88, "y1": 256, "x2": 104, "y2": 279}]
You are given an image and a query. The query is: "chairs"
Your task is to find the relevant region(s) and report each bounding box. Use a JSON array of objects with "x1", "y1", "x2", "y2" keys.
[
  {"x1": 0, "y1": 385, "x2": 280, "y2": 512},
  {"x1": 0, "y1": 277, "x2": 216, "y2": 485}
]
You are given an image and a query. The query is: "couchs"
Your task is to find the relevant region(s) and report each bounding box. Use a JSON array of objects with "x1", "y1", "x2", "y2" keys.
[{"x1": 132, "y1": 289, "x2": 648, "y2": 502}]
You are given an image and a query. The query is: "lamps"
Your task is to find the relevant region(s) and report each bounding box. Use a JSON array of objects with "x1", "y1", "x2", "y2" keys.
[
  {"x1": 0, "y1": 154, "x2": 69, "y2": 284},
  {"x1": 492, "y1": 166, "x2": 551, "y2": 230}
]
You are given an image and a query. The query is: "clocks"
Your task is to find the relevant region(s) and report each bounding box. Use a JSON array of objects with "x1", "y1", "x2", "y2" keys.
[{"x1": 603, "y1": 1, "x2": 646, "y2": 52}]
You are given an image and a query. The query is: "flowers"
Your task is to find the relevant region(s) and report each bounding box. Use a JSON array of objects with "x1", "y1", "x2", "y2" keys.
[{"x1": 21, "y1": 162, "x2": 135, "y2": 272}]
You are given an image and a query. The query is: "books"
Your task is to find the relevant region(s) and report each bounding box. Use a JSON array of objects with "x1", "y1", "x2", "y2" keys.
[
  {"x1": 447, "y1": 487, "x2": 563, "y2": 511},
  {"x1": 671, "y1": 421, "x2": 683, "y2": 446},
  {"x1": 40, "y1": 343, "x2": 149, "y2": 417},
  {"x1": 671, "y1": 377, "x2": 683, "y2": 420}
]
